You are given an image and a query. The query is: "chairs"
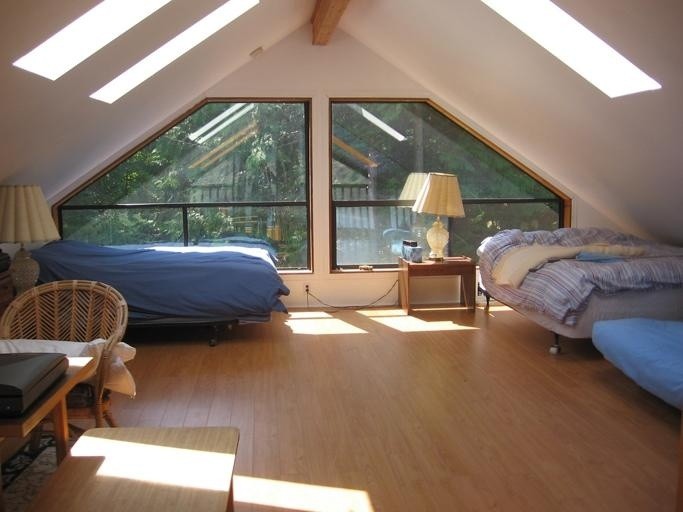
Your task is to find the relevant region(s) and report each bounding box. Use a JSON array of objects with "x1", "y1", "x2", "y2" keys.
[{"x1": 0, "y1": 279, "x2": 129, "y2": 458}]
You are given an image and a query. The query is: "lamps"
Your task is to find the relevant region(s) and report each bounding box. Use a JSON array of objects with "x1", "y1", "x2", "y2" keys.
[
  {"x1": 417, "y1": 171, "x2": 467, "y2": 261},
  {"x1": 397, "y1": 172, "x2": 427, "y2": 246},
  {"x1": 0, "y1": 183, "x2": 62, "y2": 298}
]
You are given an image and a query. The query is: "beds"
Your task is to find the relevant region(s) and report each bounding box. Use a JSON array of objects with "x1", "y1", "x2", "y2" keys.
[
  {"x1": 476, "y1": 227, "x2": 682, "y2": 356},
  {"x1": 591, "y1": 317, "x2": 683, "y2": 411},
  {"x1": 1, "y1": 240, "x2": 290, "y2": 342}
]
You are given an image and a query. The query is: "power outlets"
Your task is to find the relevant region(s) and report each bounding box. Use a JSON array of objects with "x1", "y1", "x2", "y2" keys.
[{"x1": 304, "y1": 284, "x2": 309, "y2": 292}]
